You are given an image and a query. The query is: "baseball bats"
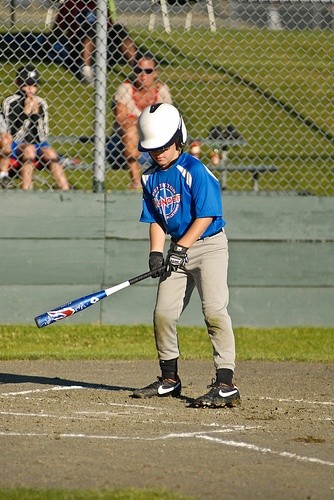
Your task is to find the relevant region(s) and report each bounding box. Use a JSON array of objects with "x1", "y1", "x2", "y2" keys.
[{"x1": 34, "y1": 256, "x2": 189, "y2": 328}]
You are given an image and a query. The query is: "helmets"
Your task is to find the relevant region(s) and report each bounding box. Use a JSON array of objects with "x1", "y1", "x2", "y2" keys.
[
  {"x1": 136, "y1": 102, "x2": 188, "y2": 171},
  {"x1": 14, "y1": 65, "x2": 42, "y2": 88}
]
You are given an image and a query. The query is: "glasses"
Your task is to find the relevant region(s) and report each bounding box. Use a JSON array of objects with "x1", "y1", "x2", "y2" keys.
[{"x1": 132, "y1": 66, "x2": 155, "y2": 75}]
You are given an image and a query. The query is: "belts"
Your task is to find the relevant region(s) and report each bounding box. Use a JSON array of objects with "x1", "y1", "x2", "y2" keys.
[{"x1": 196, "y1": 228, "x2": 223, "y2": 241}]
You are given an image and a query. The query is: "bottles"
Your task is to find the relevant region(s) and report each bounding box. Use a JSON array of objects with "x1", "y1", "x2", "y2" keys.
[
  {"x1": 210, "y1": 143, "x2": 220, "y2": 165},
  {"x1": 189, "y1": 141, "x2": 201, "y2": 159}
]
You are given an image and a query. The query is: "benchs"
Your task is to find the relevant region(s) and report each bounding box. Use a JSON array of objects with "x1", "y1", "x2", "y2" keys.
[
  {"x1": 66, "y1": 161, "x2": 280, "y2": 192},
  {"x1": 50, "y1": 136, "x2": 246, "y2": 191}
]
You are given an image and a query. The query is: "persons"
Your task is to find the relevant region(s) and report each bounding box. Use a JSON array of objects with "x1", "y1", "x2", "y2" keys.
[
  {"x1": 115, "y1": 54, "x2": 172, "y2": 188},
  {"x1": 133, "y1": 103, "x2": 241, "y2": 406},
  {"x1": 0, "y1": 65, "x2": 70, "y2": 190},
  {"x1": 55, "y1": 0, "x2": 137, "y2": 80}
]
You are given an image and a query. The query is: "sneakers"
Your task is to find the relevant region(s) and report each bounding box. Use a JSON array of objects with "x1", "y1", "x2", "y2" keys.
[
  {"x1": 190, "y1": 380, "x2": 241, "y2": 410},
  {"x1": 132, "y1": 375, "x2": 182, "y2": 400}
]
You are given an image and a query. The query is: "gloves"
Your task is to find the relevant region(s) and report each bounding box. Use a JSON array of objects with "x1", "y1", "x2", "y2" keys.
[
  {"x1": 163, "y1": 241, "x2": 190, "y2": 277},
  {"x1": 149, "y1": 252, "x2": 167, "y2": 278}
]
